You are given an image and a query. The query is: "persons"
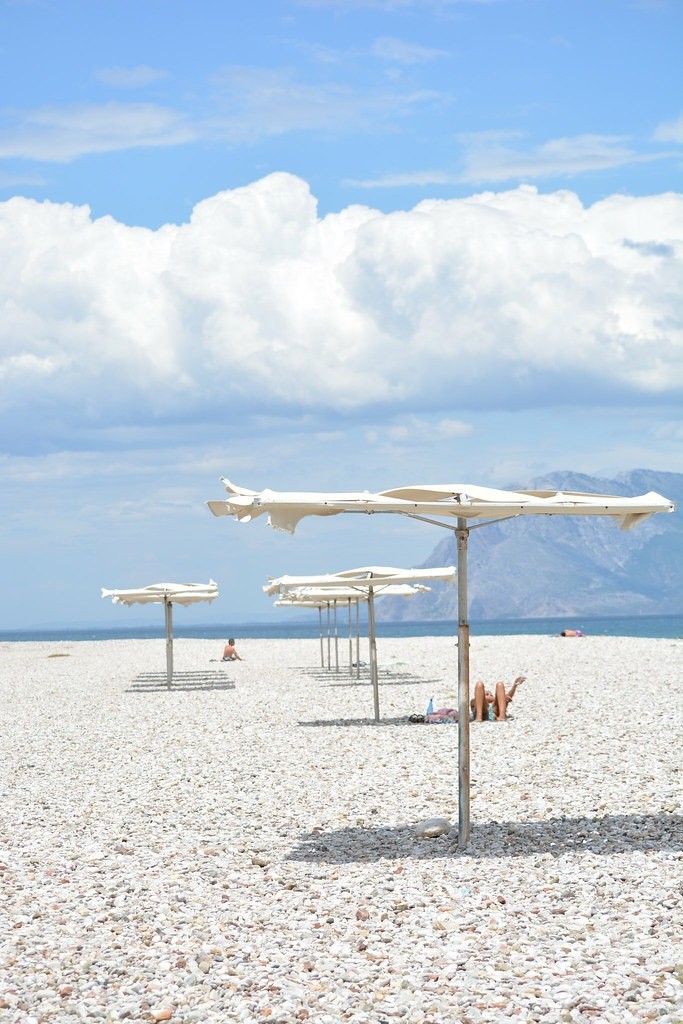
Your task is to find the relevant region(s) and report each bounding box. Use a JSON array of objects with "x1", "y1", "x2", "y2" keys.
[
  {"x1": 471, "y1": 676, "x2": 528, "y2": 723},
  {"x1": 560, "y1": 630, "x2": 582, "y2": 637},
  {"x1": 222, "y1": 639, "x2": 242, "y2": 661}
]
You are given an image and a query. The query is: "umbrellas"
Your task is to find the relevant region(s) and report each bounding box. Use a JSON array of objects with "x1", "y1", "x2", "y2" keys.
[
  {"x1": 262, "y1": 565, "x2": 456, "y2": 721},
  {"x1": 102, "y1": 579, "x2": 218, "y2": 687},
  {"x1": 202, "y1": 476, "x2": 675, "y2": 844}
]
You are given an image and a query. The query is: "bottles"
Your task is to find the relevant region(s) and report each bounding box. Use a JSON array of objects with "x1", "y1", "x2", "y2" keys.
[
  {"x1": 486, "y1": 703, "x2": 495, "y2": 721},
  {"x1": 427, "y1": 699, "x2": 433, "y2": 715}
]
